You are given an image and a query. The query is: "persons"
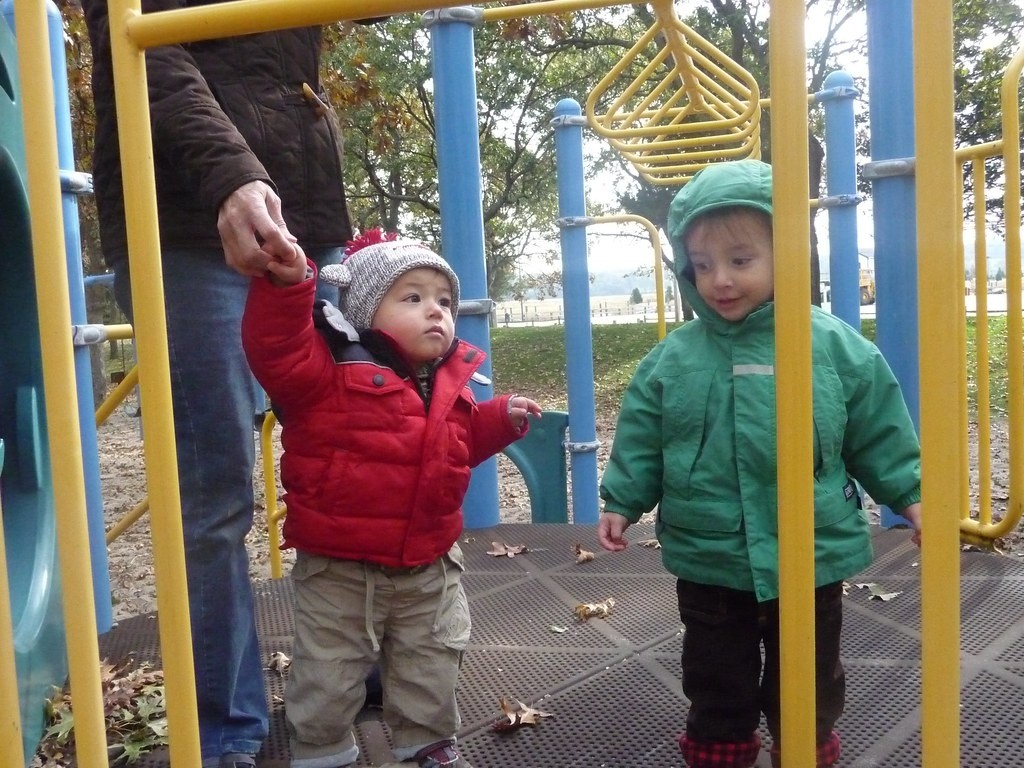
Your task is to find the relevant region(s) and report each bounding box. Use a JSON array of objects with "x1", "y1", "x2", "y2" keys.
[
  {"x1": 79, "y1": 0, "x2": 388, "y2": 758},
  {"x1": 597, "y1": 158, "x2": 920, "y2": 763},
  {"x1": 243, "y1": 230, "x2": 540, "y2": 768}
]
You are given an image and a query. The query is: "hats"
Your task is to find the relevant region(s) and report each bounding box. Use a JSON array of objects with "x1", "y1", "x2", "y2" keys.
[{"x1": 319, "y1": 230, "x2": 461, "y2": 329}]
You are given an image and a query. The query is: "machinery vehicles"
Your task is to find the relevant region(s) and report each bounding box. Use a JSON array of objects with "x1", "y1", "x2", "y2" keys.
[{"x1": 859, "y1": 269, "x2": 875, "y2": 306}]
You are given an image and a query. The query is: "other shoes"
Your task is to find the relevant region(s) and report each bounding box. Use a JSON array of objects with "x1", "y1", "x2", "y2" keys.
[
  {"x1": 419, "y1": 746, "x2": 474, "y2": 768},
  {"x1": 219, "y1": 753, "x2": 256, "y2": 768}
]
